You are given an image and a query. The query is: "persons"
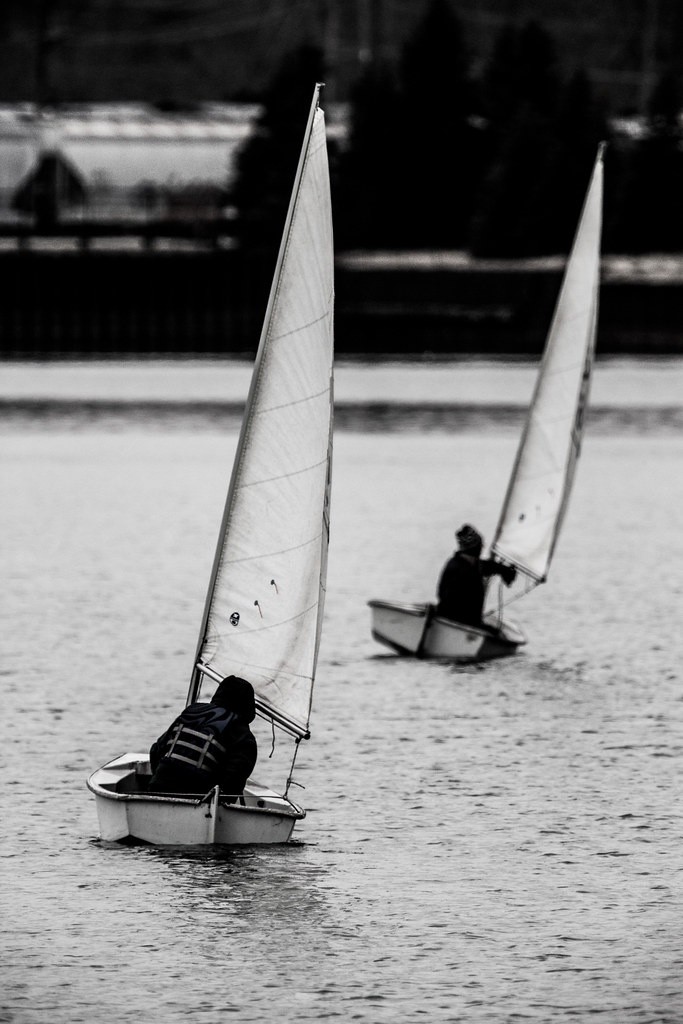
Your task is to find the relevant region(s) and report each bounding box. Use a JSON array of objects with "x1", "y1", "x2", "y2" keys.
[
  {"x1": 149, "y1": 675, "x2": 258, "y2": 805},
  {"x1": 437, "y1": 525, "x2": 516, "y2": 627}
]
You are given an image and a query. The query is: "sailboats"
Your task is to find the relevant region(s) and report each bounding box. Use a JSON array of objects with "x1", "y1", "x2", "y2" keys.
[
  {"x1": 366, "y1": 138, "x2": 607, "y2": 666},
  {"x1": 85, "y1": 80, "x2": 337, "y2": 853}
]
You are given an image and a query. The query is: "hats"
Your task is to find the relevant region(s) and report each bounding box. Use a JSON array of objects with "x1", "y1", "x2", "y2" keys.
[{"x1": 455, "y1": 526, "x2": 481, "y2": 550}]
